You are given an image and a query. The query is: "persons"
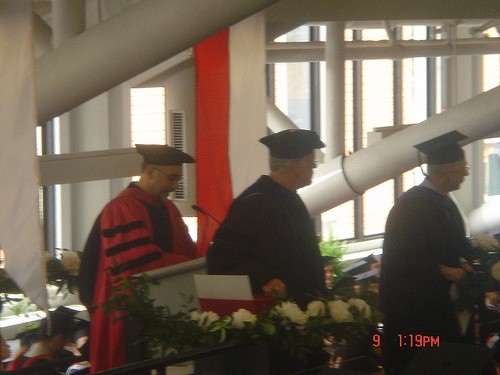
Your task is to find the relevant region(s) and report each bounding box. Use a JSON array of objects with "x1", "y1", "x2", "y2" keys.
[
  {"x1": 379, "y1": 130, "x2": 500, "y2": 375},
  {"x1": 194, "y1": 129, "x2": 327, "y2": 375},
  {"x1": 79, "y1": 144, "x2": 197, "y2": 375},
  {"x1": 321, "y1": 254, "x2": 380, "y2": 293},
  {"x1": 0, "y1": 306, "x2": 91, "y2": 375}
]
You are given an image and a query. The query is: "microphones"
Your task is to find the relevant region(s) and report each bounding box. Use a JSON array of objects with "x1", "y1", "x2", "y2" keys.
[{"x1": 192, "y1": 205, "x2": 220, "y2": 225}]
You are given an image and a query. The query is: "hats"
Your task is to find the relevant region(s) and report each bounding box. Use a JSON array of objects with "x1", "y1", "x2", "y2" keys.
[
  {"x1": 322, "y1": 254, "x2": 378, "y2": 291},
  {"x1": 16, "y1": 306, "x2": 91, "y2": 352},
  {"x1": 136, "y1": 144, "x2": 196, "y2": 165},
  {"x1": 260, "y1": 129, "x2": 325, "y2": 158},
  {"x1": 414, "y1": 130, "x2": 470, "y2": 180}
]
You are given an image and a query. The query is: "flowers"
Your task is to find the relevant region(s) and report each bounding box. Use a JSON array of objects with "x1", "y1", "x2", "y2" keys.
[
  {"x1": 470, "y1": 232, "x2": 500, "y2": 282},
  {"x1": 44, "y1": 248, "x2": 83, "y2": 296},
  {"x1": 98, "y1": 258, "x2": 375, "y2": 364}
]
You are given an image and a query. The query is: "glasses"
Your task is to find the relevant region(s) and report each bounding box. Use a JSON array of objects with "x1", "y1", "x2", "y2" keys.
[{"x1": 155, "y1": 168, "x2": 186, "y2": 182}]
